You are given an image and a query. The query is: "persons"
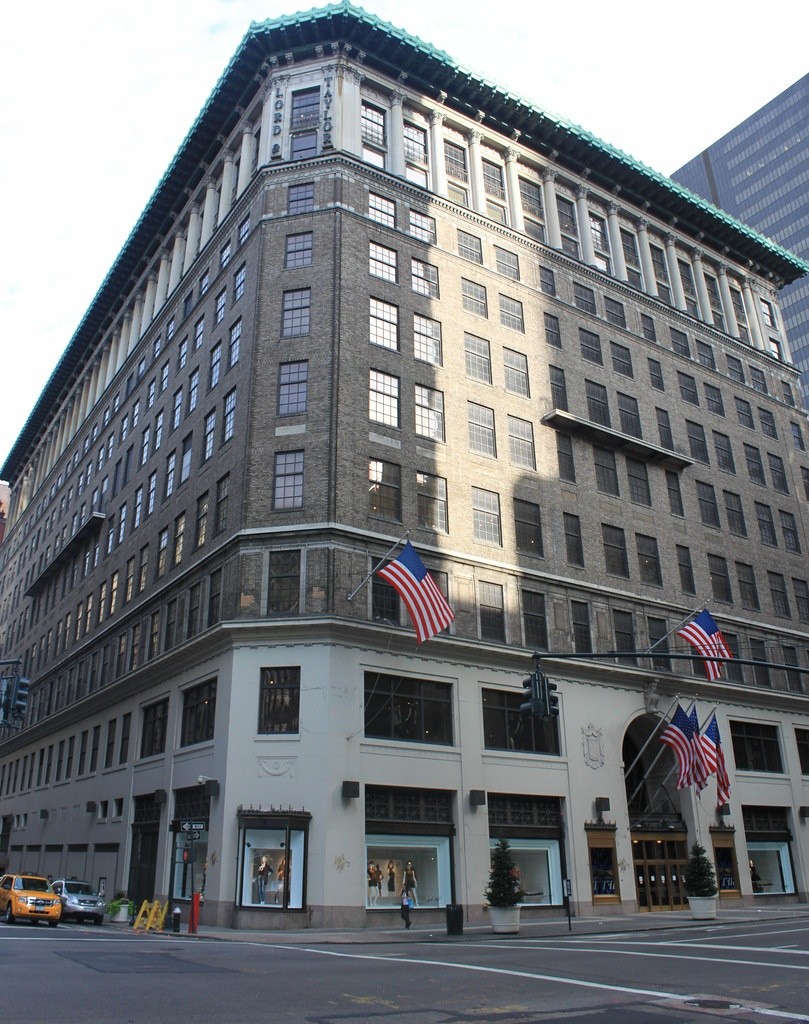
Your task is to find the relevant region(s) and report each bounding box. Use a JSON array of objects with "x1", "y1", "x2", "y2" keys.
[
  {"x1": 367, "y1": 860, "x2": 420, "y2": 907},
  {"x1": 256, "y1": 856, "x2": 273, "y2": 904},
  {"x1": 399, "y1": 884, "x2": 413, "y2": 930},
  {"x1": 275, "y1": 857, "x2": 291, "y2": 905}
]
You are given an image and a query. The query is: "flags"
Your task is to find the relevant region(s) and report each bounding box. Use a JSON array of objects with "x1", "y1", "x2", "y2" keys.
[
  {"x1": 659, "y1": 700, "x2": 711, "y2": 791},
  {"x1": 675, "y1": 608, "x2": 733, "y2": 683},
  {"x1": 687, "y1": 702, "x2": 732, "y2": 807},
  {"x1": 373, "y1": 534, "x2": 456, "y2": 645}
]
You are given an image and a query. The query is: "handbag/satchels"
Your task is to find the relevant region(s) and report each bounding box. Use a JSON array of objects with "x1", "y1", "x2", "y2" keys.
[{"x1": 408, "y1": 899, "x2": 414, "y2": 909}]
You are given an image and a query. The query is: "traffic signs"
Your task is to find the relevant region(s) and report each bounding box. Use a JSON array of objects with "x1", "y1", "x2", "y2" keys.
[
  {"x1": 179, "y1": 821, "x2": 207, "y2": 831},
  {"x1": 186, "y1": 832, "x2": 201, "y2": 840}
]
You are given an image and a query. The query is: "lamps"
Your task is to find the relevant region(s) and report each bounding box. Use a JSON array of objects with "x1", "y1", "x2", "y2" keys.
[
  {"x1": 342, "y1": 781, "x2": 359, "y2": 797},
  {"x1": 86, "y1": 801, "x2": 97, "y2": 813},
  {"x1": 41, "y1": 809, "x2": 49, "y2": 819},
  {"x1": 280, "y1": 842, "x2": 286, "y2": 847},
  {"x1": 800, "y1": 806, "x2": 809, "y2": 817},
  {"x1": 6, "y1": 816, "x2": 15, "y2": 824},
  {"x1": 245, "y1": 842, "x2": 251, "y2": 847},
  {"x1": 720, "y1": 803, "x2": 730, "y2": 815},
  {"x1": 154, "y1": 790, "x2": 166, "y2": 803},
  {"x1": 205, "y1": 780, "x2": 218, "y2": 796},
  {"x1": 596, "y1": 797, "x2": 610, "y2": 811},
  {"x1": 470, "y1": 790, "x2": 486, "y2": 805}
]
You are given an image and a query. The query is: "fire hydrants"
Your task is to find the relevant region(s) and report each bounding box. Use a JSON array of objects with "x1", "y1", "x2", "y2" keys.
[{"x1": 172, "y1": 907, "x2": 182, "y2": 933}]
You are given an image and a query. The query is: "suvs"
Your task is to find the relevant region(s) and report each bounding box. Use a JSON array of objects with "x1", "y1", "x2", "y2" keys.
[
  {"x1": 46, "y1": 878, "x2": 106, "y2": 925},
  {"x1": 0, "y1": 874, "x2": 62, "y2": 927}
]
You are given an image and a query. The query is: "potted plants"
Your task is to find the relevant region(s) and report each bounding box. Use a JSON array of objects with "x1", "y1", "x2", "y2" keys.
[
  {"x1": 487, "y1": 834, "x2": 525, "y2": 933},
  {"x1": 684, "y1": 841, "x2": 720, "y2": 920},
  {"x1": 104, "y1": 891, "x2": 137, "y2": 922}
]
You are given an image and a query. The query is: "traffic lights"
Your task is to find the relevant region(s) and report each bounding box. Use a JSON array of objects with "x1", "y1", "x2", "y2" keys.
[
  {"x1": 7, "y1": 675, "x2": 30, "y2": 721},
  {"x1": 182, "y1": 847, "x2": 197, "y2": 863},
  {"x1": 519, "y1": 671, "x2": 559, "y2": 719}
]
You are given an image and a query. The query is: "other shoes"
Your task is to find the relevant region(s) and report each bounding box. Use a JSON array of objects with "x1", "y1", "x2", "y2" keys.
[{"x1": 405, "y1": 922, "x2": 412, "y2": 928}]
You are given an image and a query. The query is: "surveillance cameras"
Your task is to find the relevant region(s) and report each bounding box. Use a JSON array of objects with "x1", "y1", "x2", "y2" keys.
[{"x1": 197, "y1": 777, "x2": 203, "y2": 784}]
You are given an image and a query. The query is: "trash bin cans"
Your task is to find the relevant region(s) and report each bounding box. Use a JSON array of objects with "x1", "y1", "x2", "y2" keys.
[{"x1": 446, "y1": 904, "x2": 463, "y2": 935}]
